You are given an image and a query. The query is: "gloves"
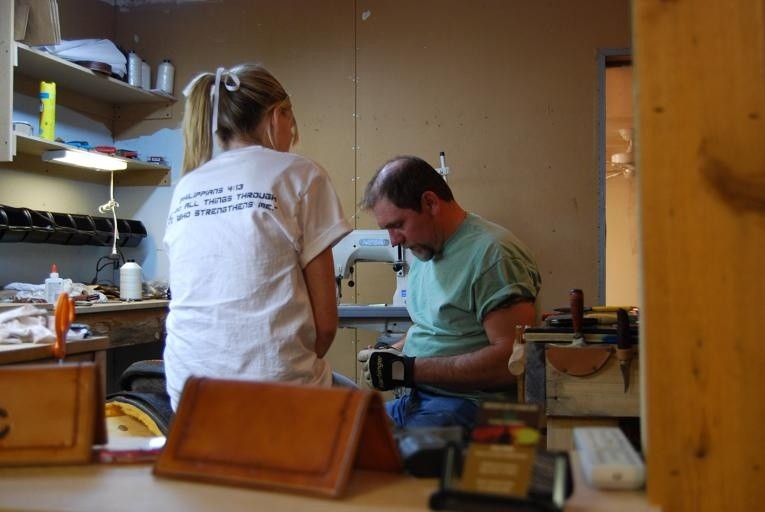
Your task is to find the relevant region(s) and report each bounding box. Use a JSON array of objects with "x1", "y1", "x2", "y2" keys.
[{"x1": 358, "y1": 344, "x2": 416, "y2": 391}]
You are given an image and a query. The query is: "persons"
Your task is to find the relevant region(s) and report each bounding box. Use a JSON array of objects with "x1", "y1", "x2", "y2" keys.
[
  {"x1": 358, "y1": 157, "x2": 542, "y2": 426},
  {"x1": 162, "y1": 65, "x2": 356, "y2": 414}
]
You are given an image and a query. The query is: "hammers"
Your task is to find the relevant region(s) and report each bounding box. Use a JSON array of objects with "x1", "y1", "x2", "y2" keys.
[{"x1": 509, "y1": 325, "x2": 527, "y2": 404}]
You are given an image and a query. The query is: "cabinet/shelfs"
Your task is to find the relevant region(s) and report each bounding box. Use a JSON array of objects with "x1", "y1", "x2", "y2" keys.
[
  {"x1": 0, "y1": 38, "x2": 173, "y2": 185},
  {"x1": 0, "y1": 338, "x2": 107, "y2": 461}
]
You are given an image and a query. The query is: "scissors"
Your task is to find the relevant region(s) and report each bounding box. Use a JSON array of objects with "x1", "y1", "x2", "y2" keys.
[{"x1": 55, "y1": 293, "x2": 76, "y2": 364}]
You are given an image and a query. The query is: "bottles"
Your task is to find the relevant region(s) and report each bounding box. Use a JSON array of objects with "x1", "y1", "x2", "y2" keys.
[
  {"x1": 45, "y1": 263, "x2": 63, "y2": 304},
  {"x1": 155, "y1": 59, "x2": 175, "y2": 95},
  {"x1": 120, "y1": 258, "x2": 143, "y2": 301},
  {"x1": 127, "y1": 49, "x2": 141, "y2": 87},
  {"x1": 141, "y1": 59, "x2": 151, "y2": 91}
]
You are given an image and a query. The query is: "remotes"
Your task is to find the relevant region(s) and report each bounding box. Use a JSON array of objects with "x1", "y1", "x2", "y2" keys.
[{"x1": 574, "y1": 425, "x2": 644, "y2": 489}]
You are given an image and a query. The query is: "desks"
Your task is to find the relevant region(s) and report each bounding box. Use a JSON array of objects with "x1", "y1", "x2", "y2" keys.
[{"x1": 28, "y1": 286, "x2": 169, "y2": 345}]
